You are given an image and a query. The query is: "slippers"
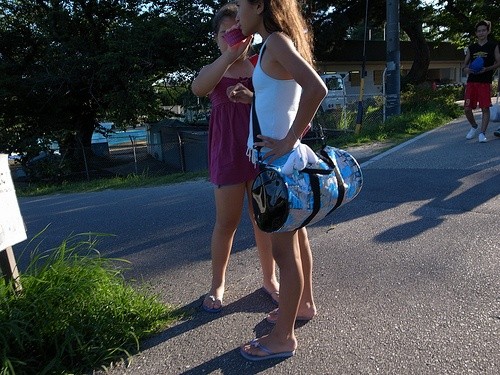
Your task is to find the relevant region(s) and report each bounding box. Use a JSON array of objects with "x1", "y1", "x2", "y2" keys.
[
  {"x1": 201, "y1": 289, "x2": 223, "y2": 312},
  {"x1": 240, "y1": 336, "x2": 295, "y2": 361},
  {"x1": 263, "y1": 287, "x2": 280, "y2": 306},
  {"x1": 267, "y1": 307, "x2": 314, "y2": 323}
]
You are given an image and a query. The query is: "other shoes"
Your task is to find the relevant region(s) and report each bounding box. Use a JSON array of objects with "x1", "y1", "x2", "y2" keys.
[{"x1": 493, "y1": 129, "x2": 500, "y2": 137}]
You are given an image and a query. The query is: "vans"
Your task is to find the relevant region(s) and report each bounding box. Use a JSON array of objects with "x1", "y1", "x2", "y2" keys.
[{"x1": 315, "y1": 74, "x2": 347, "y2": 119}]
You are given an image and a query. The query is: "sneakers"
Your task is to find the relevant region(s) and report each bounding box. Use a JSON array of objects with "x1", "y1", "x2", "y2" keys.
[
  {"x1": 466, "y1": 124, "x2": 481, "y2": 140},
  {"x1": 479, "y1": 132, "x2": 488, "y2": 143}
]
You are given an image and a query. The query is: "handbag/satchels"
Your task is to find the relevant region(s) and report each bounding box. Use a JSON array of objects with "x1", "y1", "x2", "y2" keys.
[{"x1": 250, "y1": 144, "x2": 363, "y2": 234}]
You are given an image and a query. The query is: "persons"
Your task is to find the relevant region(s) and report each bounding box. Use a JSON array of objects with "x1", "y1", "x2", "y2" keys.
[
  {"x1": 191, "y1": 2, "x2": 281, "y2": 313},
  {"x1": 227, "y1": 0, "x2": 329, "y2": 362},
  {"x1": 461, "y1": 19, "x2": 500, "y2": 143}
]
